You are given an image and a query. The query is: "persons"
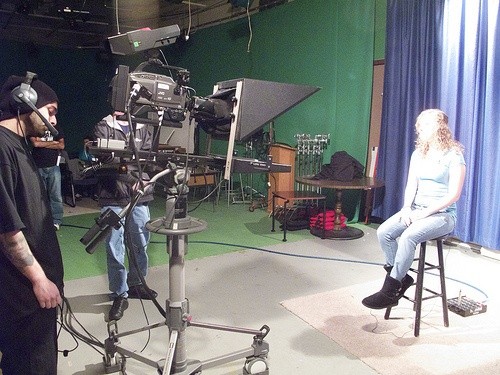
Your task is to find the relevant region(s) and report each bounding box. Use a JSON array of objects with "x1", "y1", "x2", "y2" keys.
[
  {"x1": 92, "y1": 107, "x2": 159, "y2": 322},
  {"x1": 29, "y1": 124, "x2": 65, "y2": 230},
  {"x1": 362, "y1": 109, "x2": 467, "y2": 310},
  {"x1": 74, "y1": 137, "x2": 99, "y2": 200},
  {"x1": 0, "y1": 75, "x2": 64, "y2": 375}
]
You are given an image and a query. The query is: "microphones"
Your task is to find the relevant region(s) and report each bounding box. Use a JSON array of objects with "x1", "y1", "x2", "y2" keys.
[{"x1": 22, "y1": 97, "x2": 59, "y2": 137}]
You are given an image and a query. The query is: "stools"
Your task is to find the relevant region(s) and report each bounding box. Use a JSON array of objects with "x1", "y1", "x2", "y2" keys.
[
  {"x1": 385, "y1": 233, "x2": 451, "y2": 338},
  {"x1": 271, "y1": 191, "x2": 327, "y2": 242}
]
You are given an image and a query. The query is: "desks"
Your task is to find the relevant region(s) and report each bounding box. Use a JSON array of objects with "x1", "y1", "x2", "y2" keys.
[{"x1": 295, "y1": 174, "x2": 384, "y2": 240}]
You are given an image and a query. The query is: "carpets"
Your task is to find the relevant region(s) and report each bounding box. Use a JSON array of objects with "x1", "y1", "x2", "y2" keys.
[{"x1": 280, "y1": 278, "x2": 500, "y2": 374}]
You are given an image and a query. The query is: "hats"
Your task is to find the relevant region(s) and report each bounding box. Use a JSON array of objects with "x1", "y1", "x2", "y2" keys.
[{"x1": 0, "y1": 74, "x2": 60, "y2": 122}]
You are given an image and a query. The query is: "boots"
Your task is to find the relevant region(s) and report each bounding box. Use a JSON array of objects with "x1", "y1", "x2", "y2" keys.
[
  {"x1": 362, "y1": 273, "x2": 405, "y2": 309},
  {"x1": 383, "y1": 265, "x2": 415, "y2": 299}
]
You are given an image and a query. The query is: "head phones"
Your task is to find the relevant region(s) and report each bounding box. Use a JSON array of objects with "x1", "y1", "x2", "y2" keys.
[{"x1": 9, "y1": 71, "x2": 39, "y2": 113}]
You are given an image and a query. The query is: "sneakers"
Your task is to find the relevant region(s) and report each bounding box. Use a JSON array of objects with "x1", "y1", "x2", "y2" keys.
[
  {"x1": 127, "y1": 284, "x2": 157, "y2": 299},
  {"x1": 109, "y1": 298, "x2": 129, "y2": 320}
]
